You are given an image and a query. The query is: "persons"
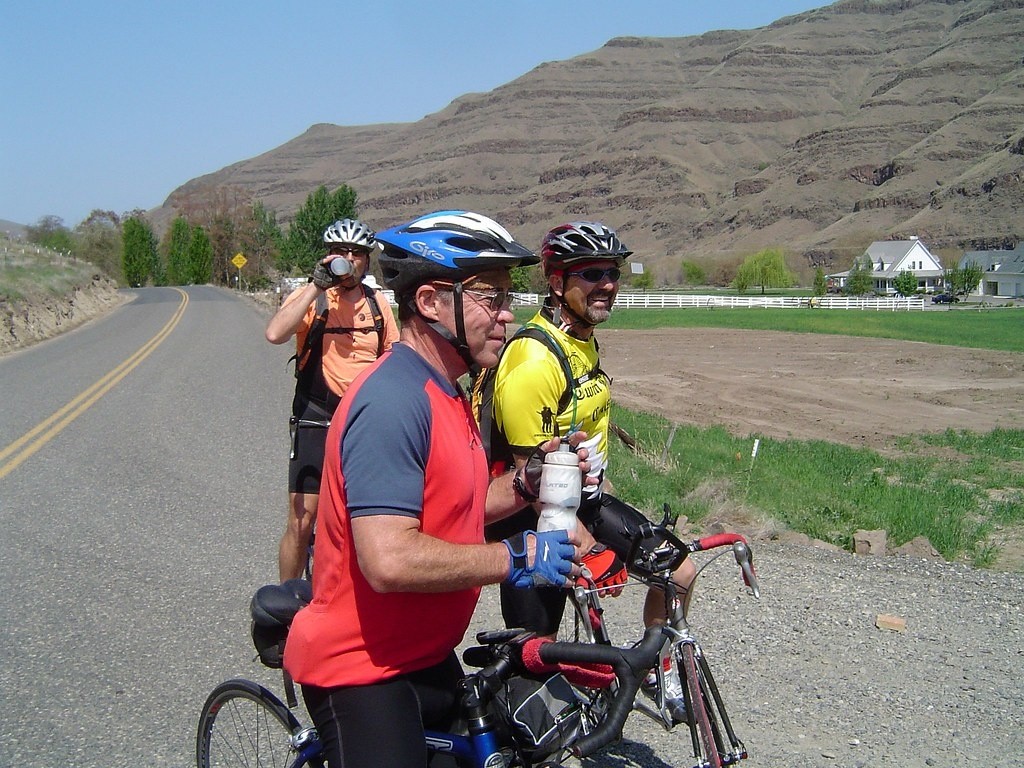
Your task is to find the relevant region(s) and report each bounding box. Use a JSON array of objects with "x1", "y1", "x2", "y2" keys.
[
  {"x1": 266, "y1": 217, "x2": 402, "y2": 586},
  {"x1": 284, "y1": 210, "x2": 599, "y2": 768},
  {"x1": 485, "y1": 222, "x2": 697, "y2": 642}
]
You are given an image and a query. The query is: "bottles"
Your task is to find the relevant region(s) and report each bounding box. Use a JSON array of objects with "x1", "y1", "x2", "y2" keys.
[
  {"x1": 534, "y1": 438, "x2": 581, "y2": 586},
  {"x1": 643, "y1": 637, "x2": 671, "y2": 690},
  {"x1": 331, "y1": 258, "x2": 356, "y2": 276}
]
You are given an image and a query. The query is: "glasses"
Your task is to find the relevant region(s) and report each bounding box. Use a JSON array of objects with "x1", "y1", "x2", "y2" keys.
[
  {"x1": 330, "y1": 246, "x2": 370, "y2": 257},
  {"x1": 561, "y1": 267, "x2": 621, "y2": 283},
  {"x1": 431, "y1": 285, "x2": 514, "y2": 311}
]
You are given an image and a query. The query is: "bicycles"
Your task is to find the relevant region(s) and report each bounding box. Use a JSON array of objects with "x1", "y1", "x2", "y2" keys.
[{"x1": 196, "y1": 532, "x2": 763, "y2": 768}]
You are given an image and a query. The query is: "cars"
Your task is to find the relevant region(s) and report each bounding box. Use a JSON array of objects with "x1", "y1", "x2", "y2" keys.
[
  {"x1": 927, "y1": 287, "x2": 951, "y2": 294},
  {"x1": 933, "y1": 294, "x2": 960, "y2": 304},
  {"x1": 826, "y1": 286, "x2": 833, "y2": 293},
  {"x1": 884, "y1": 288, "x2": 899, "y2": 297}
]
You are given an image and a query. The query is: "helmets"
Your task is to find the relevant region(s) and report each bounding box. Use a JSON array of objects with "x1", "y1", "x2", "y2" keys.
[
  {"x1": 376, "y1": 210, "x2": 540, "y2": 305},
  {"x1": 324, "y1": 218, "x2": 378, "y2": 253},
  {"x1": 541, "y1": 220, "x2": 634, "y2": 277}
]
